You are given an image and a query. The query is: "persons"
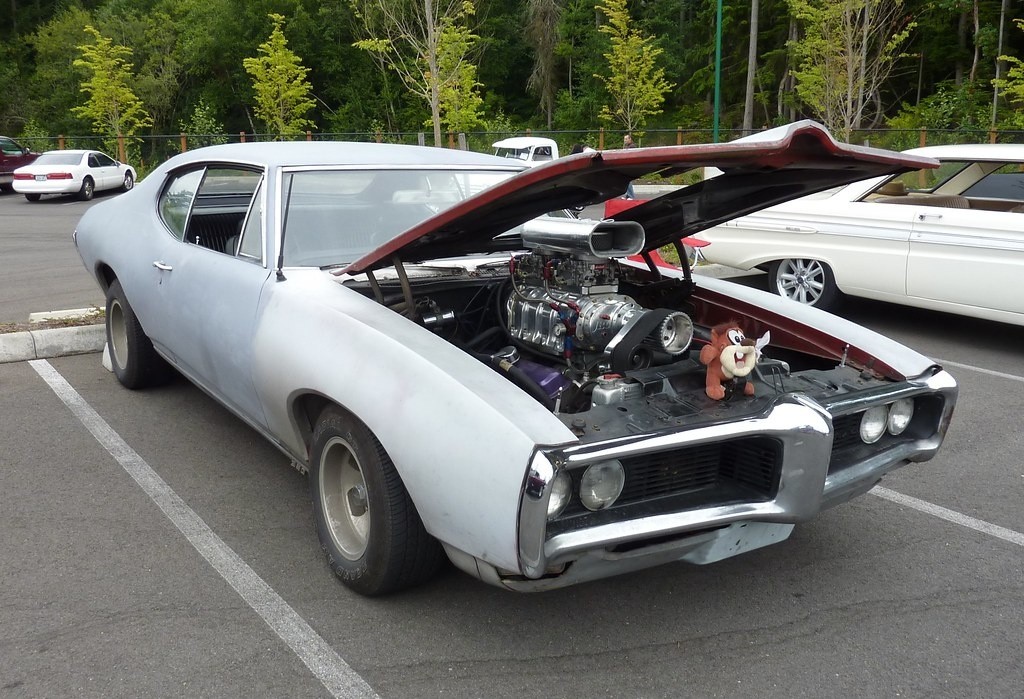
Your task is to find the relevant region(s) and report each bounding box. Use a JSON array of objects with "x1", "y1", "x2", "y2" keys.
[
  {"x1": 569, "y1": 142, "x2": 598, "y2": 154},
  {"x1": 524, "y1": 127, "x2": 532, "y2": 136},
  {"x1": 621, "y1": 135, "x2": 639, "y2": 201}
]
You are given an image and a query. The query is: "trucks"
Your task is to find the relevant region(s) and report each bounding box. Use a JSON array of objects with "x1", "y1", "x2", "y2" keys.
[{"x1": 494, "y1": 137, "x2": 561, "y2": 168}]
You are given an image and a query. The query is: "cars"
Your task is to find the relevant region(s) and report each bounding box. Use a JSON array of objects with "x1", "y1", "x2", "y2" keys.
[
  {"x1": 0, "y1": 134, "x2": 42, "y2": 193},
  {"x1": 73, "y1": 117, "x2": 958, "y2": 591},
  {"x1": 12, "y1": 149, "x2": 138, "y2": 202},
  {"x1": 685, "y1": 142, "x2": 1024, "y2": 337}
]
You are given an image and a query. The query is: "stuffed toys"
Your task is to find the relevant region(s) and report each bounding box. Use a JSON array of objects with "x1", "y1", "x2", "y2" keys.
[{"x1": 699, "y1": 322, "x2": 756, "y2": 400}]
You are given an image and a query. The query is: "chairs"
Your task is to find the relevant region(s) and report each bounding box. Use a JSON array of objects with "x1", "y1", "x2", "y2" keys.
[{"x1": 226, "y1": 216, "x2": 299, "y2": 264}]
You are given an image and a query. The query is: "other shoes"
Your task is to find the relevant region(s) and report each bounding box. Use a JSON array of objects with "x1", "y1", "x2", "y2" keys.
[{"x1": 620, "y1": 195, "x2": 634, "y2": 200}]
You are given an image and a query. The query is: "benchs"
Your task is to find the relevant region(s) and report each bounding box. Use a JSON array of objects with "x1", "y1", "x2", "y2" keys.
[
  {"x1": 180, "y1": 205, "x2": 430, "y2": 257},
  {"x1": 873, "y1": 194, "x2": 971, "y2": 209}
]
